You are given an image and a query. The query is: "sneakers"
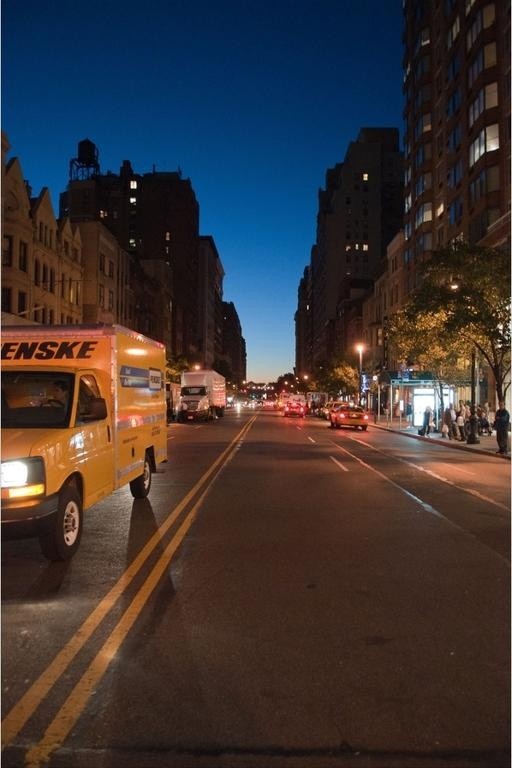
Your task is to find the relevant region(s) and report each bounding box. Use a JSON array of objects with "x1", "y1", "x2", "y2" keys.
[{"x1": 496, "y1": 449, "x2": 507, "y2": 454}]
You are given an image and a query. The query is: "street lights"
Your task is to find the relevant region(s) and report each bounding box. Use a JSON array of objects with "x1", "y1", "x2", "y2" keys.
[
  {"x1": 355, "y1": 343, "x2": 365, "y2": 374},
  {"x1": 451, "y1": 281, "x2": 483, "y2": 445}
]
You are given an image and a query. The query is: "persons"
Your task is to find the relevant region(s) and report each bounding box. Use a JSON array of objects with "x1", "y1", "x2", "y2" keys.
[
  {"x1": 416, "y1": 397, "x2": 510, "y2": 456},
  {"x1": 37, "y1": 379, "x2": 70, "y2": 409}
]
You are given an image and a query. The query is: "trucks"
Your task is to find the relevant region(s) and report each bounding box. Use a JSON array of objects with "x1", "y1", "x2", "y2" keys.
[{"x1": 3, "y1": 323, "x2": 168, "y2": 560}]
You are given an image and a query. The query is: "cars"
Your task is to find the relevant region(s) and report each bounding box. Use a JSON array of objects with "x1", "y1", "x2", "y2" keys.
[{"x1": 166, "y1": 369, "x2": 370, "y2": 432}]
